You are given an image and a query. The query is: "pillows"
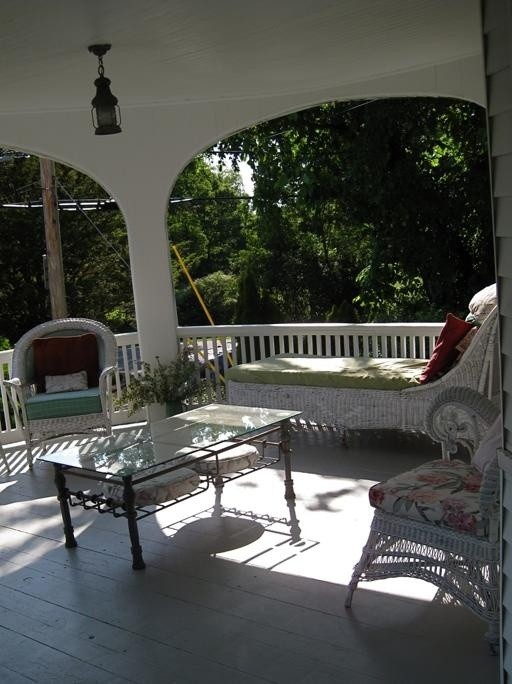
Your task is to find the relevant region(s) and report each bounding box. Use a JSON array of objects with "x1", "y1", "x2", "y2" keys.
[
  {"x1": 417, "y1": 312, "x2": 471, "y2": 388},
  {"x1": 471, "y1": 411, "x2": 501, "y2": 472},
  {"x1": 42, "y1": 369, "x2": 90, "y2": 393},
  {"x1": 28, "y1": 331, "x2": 99, "y2": 395}
]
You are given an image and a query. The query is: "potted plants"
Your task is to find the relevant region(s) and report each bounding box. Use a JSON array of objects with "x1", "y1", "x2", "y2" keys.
[{"x1": 111, "y1": 344, "x2": 218, "y2": 419}]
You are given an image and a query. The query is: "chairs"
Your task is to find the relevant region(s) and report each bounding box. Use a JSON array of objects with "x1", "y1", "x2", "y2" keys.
[
  {"x1": 2, "y1": 316, "x2": 119, "y2": 470},
  {"x1": 343, "y1": 384, "x2": 502, "y2": 655}
]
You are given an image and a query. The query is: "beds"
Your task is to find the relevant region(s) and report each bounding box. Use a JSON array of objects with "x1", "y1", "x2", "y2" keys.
[{"x1": 225, "y1": 277, "x2": 498, "y2": 460}]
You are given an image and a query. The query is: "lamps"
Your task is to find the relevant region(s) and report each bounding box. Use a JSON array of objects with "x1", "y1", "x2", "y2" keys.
[{"x1": 86, "y1": 41, "x2": 124, "y2": 137}]
